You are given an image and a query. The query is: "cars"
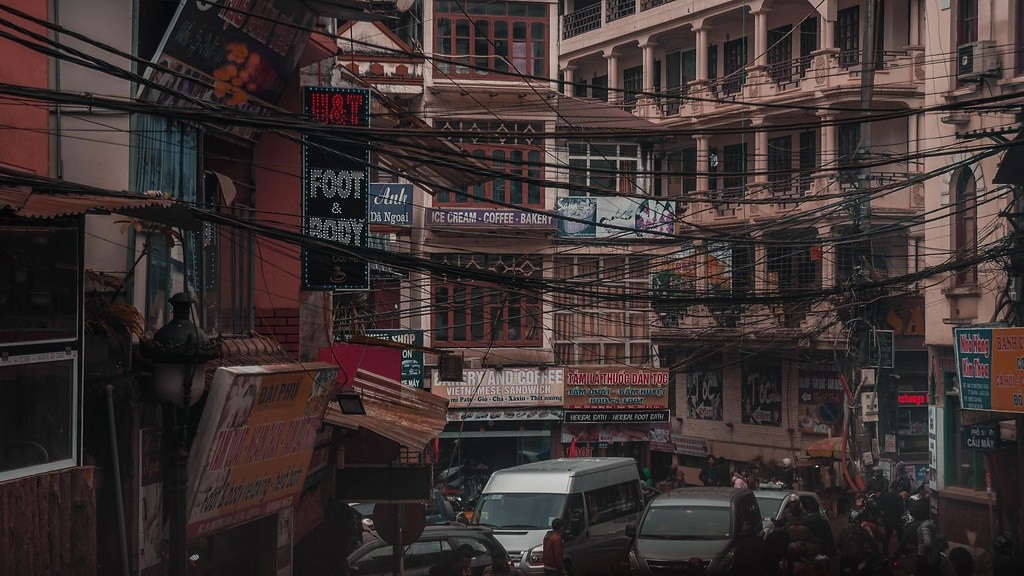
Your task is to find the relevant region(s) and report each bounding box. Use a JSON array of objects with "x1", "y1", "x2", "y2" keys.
[{"x1": 347, "y1": 528, "x2": 519, "y2": 576}]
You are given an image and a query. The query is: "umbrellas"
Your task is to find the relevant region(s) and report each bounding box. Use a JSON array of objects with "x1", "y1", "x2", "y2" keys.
[{"x1": 802, "y1": 436, "x2": 880, "y2": 463}]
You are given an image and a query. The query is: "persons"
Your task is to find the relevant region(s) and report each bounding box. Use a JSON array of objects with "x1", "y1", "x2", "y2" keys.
[
  {"x1": 699, "y1": 454, "x2": 720, "y2": 486},
  {"x1": 541, "y1": 518, "x2": 567, "y2": 576},
  {"x1": 432, "y1": 544, "x2": 474, "y2": 576},
  {"x1": 716, "y1": 456, "x2": 729, "y2": 486},
  {"x1": 861, "y1": 463, "x2": 979, "y2": 576},
  {"x1": 732, "y1": 472, "x2": 748, "y2": 489}
]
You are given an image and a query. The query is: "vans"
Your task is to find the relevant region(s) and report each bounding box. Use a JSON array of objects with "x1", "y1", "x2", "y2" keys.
[
  {"x1": 752, "y1": 489, "x2": 825, "y2": 559},
  {"x1": 623, "y1": 485, "x2": 764, "y2": 576},
  {"x1": 468, "y1": 455, "x2": 644, "y2": 576}
]
